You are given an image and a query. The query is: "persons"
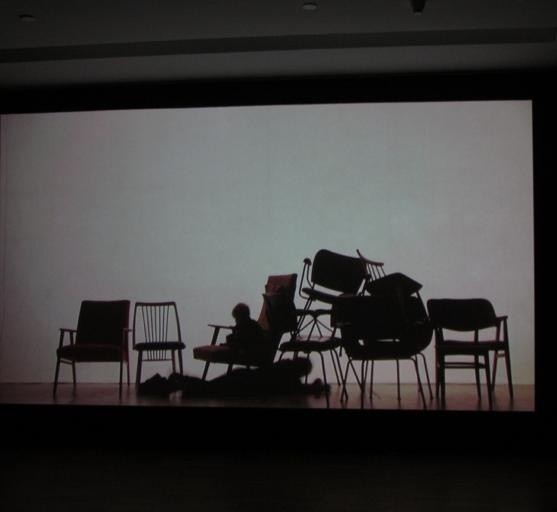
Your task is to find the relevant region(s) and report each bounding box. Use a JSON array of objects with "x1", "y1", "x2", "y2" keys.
[
  {"x1": 136, "y1": 356, "x2": 324, "y2": 399},
  {"x1": 218, "y1": 300, "x2": 263, "y2": 350}
]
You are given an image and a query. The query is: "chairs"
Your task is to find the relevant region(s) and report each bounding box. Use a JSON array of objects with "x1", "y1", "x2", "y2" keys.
[
  {"x1": 52, "y1": 297, "x2": 133, "y2": 403},
  {"x1": 193, "y1": 247, "x2": 512, "y2": 410},
  {"x1": 130, "y1": 300, "x2": 187, "y2": 377}
]
